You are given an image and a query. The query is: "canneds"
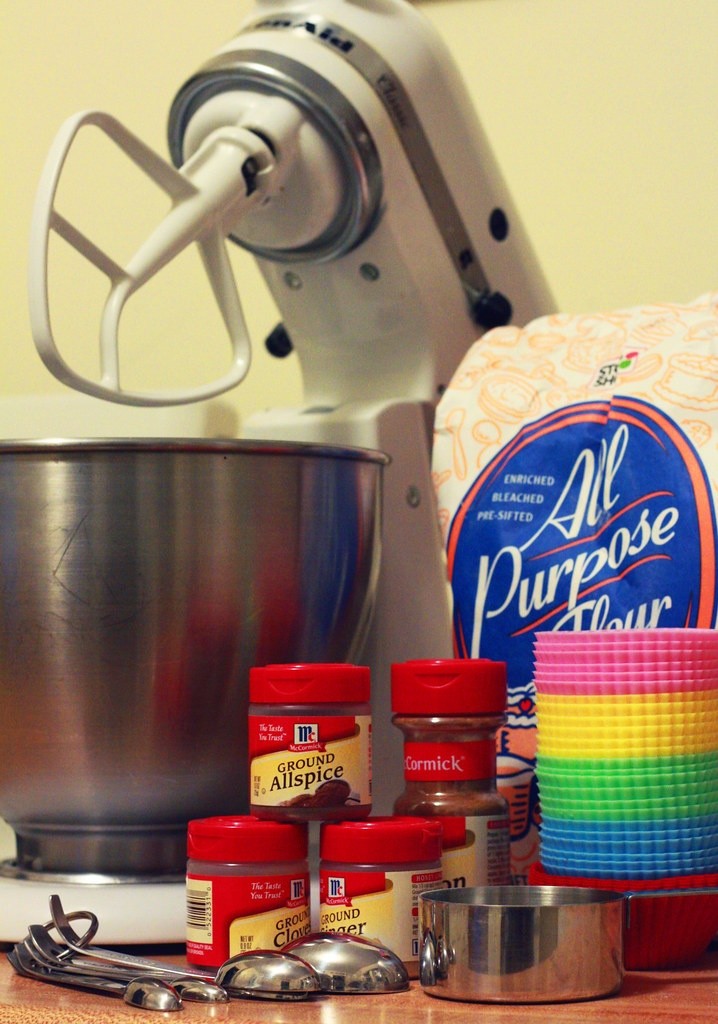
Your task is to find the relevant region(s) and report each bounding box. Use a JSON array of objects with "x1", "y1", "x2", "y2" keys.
[{"x1": 186, "y1": 657, "x2": 513, "y2": 981}]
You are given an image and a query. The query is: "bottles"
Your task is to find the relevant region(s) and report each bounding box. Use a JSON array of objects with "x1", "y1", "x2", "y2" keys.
[
  {"x1": 319, "y1": 816, "x2": 443, "y2": 980},
  {"x1": 390, "y1": 659, "x2": 510, "y2": 891},
  {"x1": 185, "y1": 815, "x2": 311, "y2": 973},
  {"x1": 248, "y1": 662, "x2": 372, "y2": 821}
]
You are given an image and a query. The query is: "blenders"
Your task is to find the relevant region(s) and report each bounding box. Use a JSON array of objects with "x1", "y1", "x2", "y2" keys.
[{"x1": 0, "y1": 0, "x2": 560, "y2": 944}]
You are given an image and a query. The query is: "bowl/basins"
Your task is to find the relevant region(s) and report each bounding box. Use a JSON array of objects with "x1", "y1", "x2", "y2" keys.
[
  {"x1": 2, "y1": 437, "x2": 391, "y2": 877},
  {"x1": 528, "y1": 628, "x2": 718, "y2": 972}
]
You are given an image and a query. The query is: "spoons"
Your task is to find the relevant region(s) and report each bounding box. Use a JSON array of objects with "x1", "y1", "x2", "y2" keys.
[{"x1": 5, "y1": 894, "x2": 410, "y2": 1013}]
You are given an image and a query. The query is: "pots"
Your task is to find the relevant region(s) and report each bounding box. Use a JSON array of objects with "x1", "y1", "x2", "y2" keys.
[{"x1": 417, "y1": 885, "x2": 718, "y2": 1003}]
